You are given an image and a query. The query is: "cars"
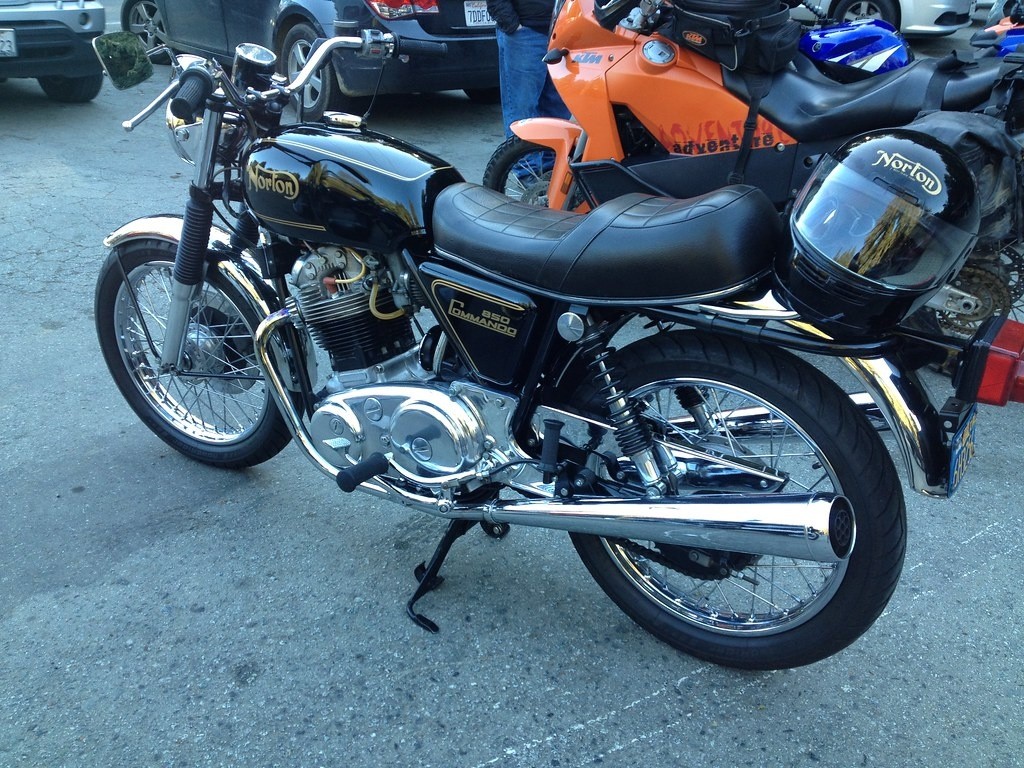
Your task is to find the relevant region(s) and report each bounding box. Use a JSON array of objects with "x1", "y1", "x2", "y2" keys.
[
  {"x1": 0, "y1": 0, "x2": 106, "y2": 104},
  {"x1": 118, "y1": 1, "x2": 501, "y2": 117}
]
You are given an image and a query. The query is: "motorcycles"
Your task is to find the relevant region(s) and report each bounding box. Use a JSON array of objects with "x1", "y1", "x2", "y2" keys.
[
  {"x1": 477, "y1": 3, "x2": 1024, "y2": 404},
  {"x1": 92, "y1": 31, "x2": 1020, "y2": 671}
]
